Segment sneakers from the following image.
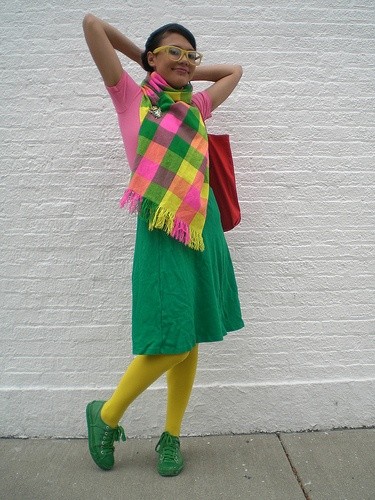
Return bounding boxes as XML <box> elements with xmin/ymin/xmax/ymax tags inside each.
<box><xmin>157</xmin><ymin>431</ymin><xmax>185</xmax><ymax>476</ymax></box>
<box><xmin>85</xmin><ymin>400</ymin><xmax>126</xmax><ymax>470</ymax></box>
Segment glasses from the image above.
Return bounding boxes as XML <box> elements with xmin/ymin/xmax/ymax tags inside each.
<box><xmin>153</xmin><ymin>45</ymin><xmax>203</xmax><ymax>66</ymax></box>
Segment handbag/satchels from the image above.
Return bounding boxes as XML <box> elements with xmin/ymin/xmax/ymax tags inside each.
<box><xmin>192</xmin><ymin>99</ymin><xmax>242</xmax><ymax>233</ymax></box>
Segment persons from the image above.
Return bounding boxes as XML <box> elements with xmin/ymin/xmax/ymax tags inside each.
<box><xmin>80</xmin><ymin>12</ymin><xmax>247</xmax><ymax>476</ymax></box>
